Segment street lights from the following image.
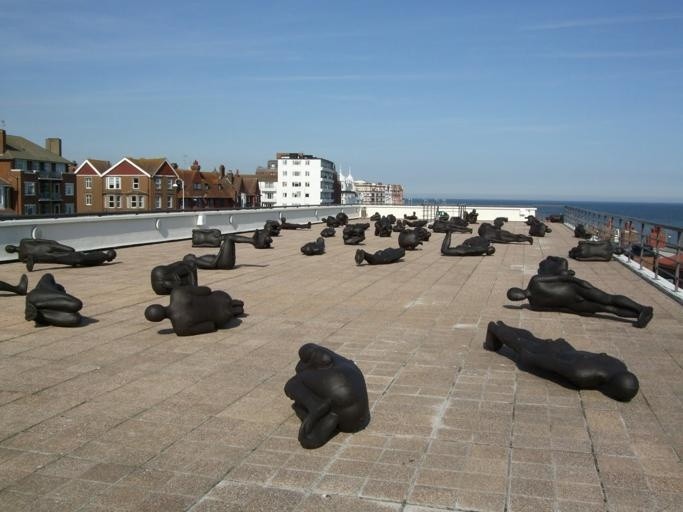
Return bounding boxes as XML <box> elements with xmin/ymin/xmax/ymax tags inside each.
<box><xmin>172</xmin><ymin>178</ymin><xmax>185</xmax><ymax>211</ymax></box>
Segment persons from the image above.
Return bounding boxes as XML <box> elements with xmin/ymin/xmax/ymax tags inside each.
<box><xmin>484</xmin><ymin>321</ymin><xmax>639</xmax><ymax>404</ymax></box>
<box><xmin>23</xmin><ymin>273</ymin><xmax>85</xmax><ymax>328</ymax></box>
<box><xmin>145</xmin><ymin>285</ymin><xmax>244</xmax><ymax>338</ymax></box>
<box><xmin>0</xmin><ymin>209</ymin><xmax>624</xmax><ymax>297</ymax></box>
<box><xmin>283</xmin><ymin>342</ymin><xmax>371</xmax><ymax>449</ymax></box>
<box><xmin>508</xmin><ymin>274</ymin><xmax>651</xmax><ymax>327</ymax></box>
